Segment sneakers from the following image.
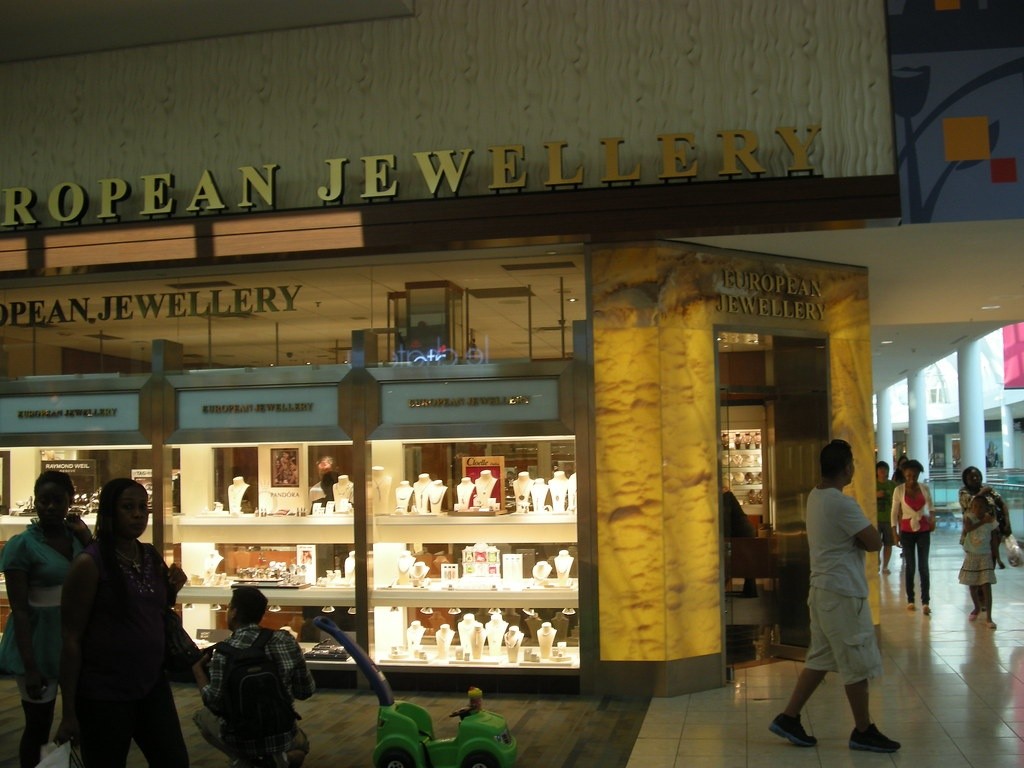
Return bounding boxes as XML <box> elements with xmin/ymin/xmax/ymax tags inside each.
<box><xmin>768</xmin><ymin>713</ymin><xmax>817</xmax><ymax>747</ymax></box>
<box><xmin>849</xmin><ymin>723</ymin><xmax>901</xmax><ymax>753</ymax></box>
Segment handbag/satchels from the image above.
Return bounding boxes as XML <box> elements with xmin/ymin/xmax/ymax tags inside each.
<box><xmin>34</xmin><ymin>736</ymin><xmax>85</xmax><ymax>768</ymax></box>
<box><xmin>990</xmin><ymin>490</ymin><xmax>1012</xmax><ymax>537</ymax></box>
<box><xmin>149</xmin><ymin>543</ymin><xmax>204</xmax><ymax>672</ymax></box>
<box><xmin>1005</xmin><ymin>534</ymin><xmax>1024</xmax><ymax>567</ymax></box>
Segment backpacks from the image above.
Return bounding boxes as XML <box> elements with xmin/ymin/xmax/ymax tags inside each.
<box><xmin>202</xmin><ymin>629</ymin><xmax>303</xmax><ymax>740</ymax></box>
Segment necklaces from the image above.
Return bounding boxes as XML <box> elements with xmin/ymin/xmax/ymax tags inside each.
<box><xmin>721</xmin><ymin>433</ymin><xmax>762</xmax><ymax>464</ymax></box>
<box><xmin>114</xmin><ymin>544</ymin><xmax>141</xmax><ymax>573</ymax></box>
<box><xmin>373</xmin><ymin>471</ymin><xmax>577</xmax><ymax>511</ymax></box>
<box><xmin>397</xmin><ymin>552</ymin><xmax>571</xmax><ymax>649</ymax></box>
<box><xmin>231</xmin><ymin>485</ymin><xmax>246</xmax><ymax>499</ymax></box>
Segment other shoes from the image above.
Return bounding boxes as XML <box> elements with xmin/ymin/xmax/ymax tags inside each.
<box><xmin>264</xmin><ymin>751</ymin><xmax>290</xmax><ymax>768</ymax></box>
<box><xmin>985</xmin><ymin>620</ymin><xmax>996</xmax><ymax>628</ymax></box>
<box><xmin>969</xmin><ymin>608</ymin><xmax>981</xmax><ymax>621</ymax></box>
<box><xmin>229</xmin><ymin>758</ymin><xmax>258</xmax><ymax>768</ymax></box>
<box><xmin>900</xmin><ymin>553</ymin><xmax>905</xmax><ymax>558</ymax></box>
<box><xmin>882</xmin><ymin>568</ymin><xmax>892</xmax><ymax>575</ymax></box>
<box><xmin>908</xmin><ymin>603</ymin><xmax>915</xmax><ymax>611</ymax></box>
<box><xmin>922</xmin><ymin>605</ymin><xmax>931</xmax><ymax>614</ymax></box>
<box><xmin>878</xmin><ymin>561</ymin><xmax>881</xmax><ymax>573</ymax></box>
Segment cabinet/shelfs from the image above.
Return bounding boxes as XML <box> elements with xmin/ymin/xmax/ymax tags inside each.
<box><xmin>0</xmin><ymin>435</ymin><xmax>580</xmax><ymax>676</ymax></box>
<box><xmin>720</xmin><ymin>427</ymin><xmax>765</xmax><ymax>513</ymax></box>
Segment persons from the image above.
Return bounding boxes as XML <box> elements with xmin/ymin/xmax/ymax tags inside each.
<box><xmin>958</xmin><ymin>495</ymin><xmax>1005</xmax><ymax>629</ymax></box>
<box><xmin>958</xmin><ymin>466</ymin><xmax>1012</xmax><ymax>611</ymax></box>
<box><xmin>767</xmin><ymin>439</ymin><xmax>902</xmax><ymax>753</ymax></box>
<box><xmin>0</xmin><ymin>469</ymin><xmax>93</xmax><ymax>768</ymax></box>
<box><xmin>191</xmin><ymin>586</ymin><xmax>315</xmax><ymax>768</ymax></box>
<box><xmin>986</xmin><ymin>441</ymin><xmax>998</xmax><ymax>468</ymax></box>
<box><xmin>876</xmin><ymin>460</ymin><xmax>899</xmax><ymax>575</ymax></box>
<box><xmin>309</xmin><ymin>473</ymin><xmax>351</xmax><ymax>578</ymax></box>
<box><xmin>892</xmin><ymin>455</ymin><xmax>909</xmax><ymax>483</ymax></box>
<box><xmin>54</xmin><ymin>477</ymin><xmax>190</xmax><ymax>768</ymax></box>
<box><xmin>722</xmin><ymin>490</ymin><xmax>754</xmax><ymax>536</ymax></box>
<box><xmin>891</xmin><ymin>459</ymin><xmax>936</xmax><ymax>614</ymax></box>
